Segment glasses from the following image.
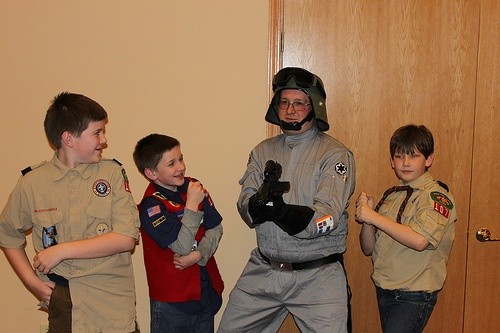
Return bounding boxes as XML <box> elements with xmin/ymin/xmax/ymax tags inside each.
<box><xmin>276</xmin><ymin>97</ymin><xmax>312</xmax><ymax>111</ymax></box>
<box><xmin>276</xmin><ymin>67</ymin><xmax>314</xmax><ymax>88</ymax></box>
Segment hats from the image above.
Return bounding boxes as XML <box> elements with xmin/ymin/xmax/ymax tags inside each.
<box><xmin>265</xmin><ymin>67</ymin><xmax>330</xmax><ymax>132</ymax></box>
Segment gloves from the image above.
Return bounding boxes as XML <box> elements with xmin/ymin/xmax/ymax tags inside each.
<box><xmin>249</xmin><ymin>160</ymin><xmax>290</xmax><ymax>222</ymax></box>
<box><xmin>253</xmin><ymin>191</ymin><xmax>315</xmax><ymax>236</ymax></box>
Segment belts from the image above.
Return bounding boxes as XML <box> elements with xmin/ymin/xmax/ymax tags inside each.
<box><xmin>258</xmin><ymin>248</ymin><xmax>342</xmax><ymax>271</ymax></box>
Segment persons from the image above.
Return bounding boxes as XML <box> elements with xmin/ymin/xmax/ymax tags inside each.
<box><xmin>355</xmin><ymin>125</ymin><xmax>456</xmax><ymax>333</ymax></box>
<box><xmin>212</xmin><ymin>68</ymin><xmax>356</xmax><ymax>333</ymax></box>
<box><xmin>0</xmin><ymin>91</ymin><xmax>140</xmax><ymax>333</ymax></box>
<box><xmin>133</xmin><ymin>134</ymin><xmax>226</xmax><ymax>333</ymax></box>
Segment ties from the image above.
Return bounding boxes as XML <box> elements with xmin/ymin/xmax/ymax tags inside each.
<box><xmin>374</xmin><ymin>185</ymin><xmax>413</xmax><ymax>226</ymax></box>
